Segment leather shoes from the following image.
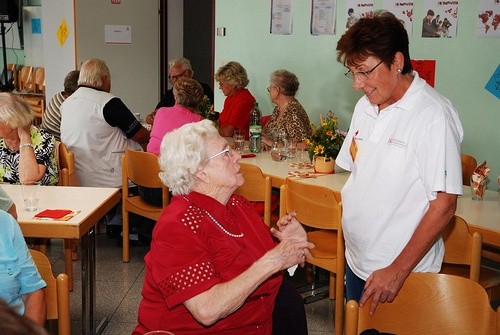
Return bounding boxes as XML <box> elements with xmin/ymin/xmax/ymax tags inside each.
<box><xmin>117</xmin><ymin>234</ymin><xmax>152</xmax><ymax>248</ymax></box>
<box><xmin>105</xmin><ymin>224</ymin><xmax>139</xmax><ymax>238</ymax></box>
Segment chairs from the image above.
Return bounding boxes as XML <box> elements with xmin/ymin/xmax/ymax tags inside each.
<box><xmin>22</xmin><ymin>249</ymin><xmax>70</xmax><ymax>335</ymax></box>
<box><xmin>121</xmin><ymin>148</ymin><xmax>169</xmax><ymax>263</ymax></box>
<box><xmin>7</xmin><ymin>64</ymin><xmax>45</xmax><ymax>127</ymax></box>
<box><xmin>56</xmin><ymin>142</ymin><xmax>78</xmax><ymax>186</ymax></box>
<box><xmin>238</xmin><ymin>163</ymin><xmax>500</xmax><ymax>335</ymax></box>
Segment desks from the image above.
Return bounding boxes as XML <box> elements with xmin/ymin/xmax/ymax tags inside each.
<box><xmin>0</xmin><ymin>184</ymin><xmax>122</xmax><ymax>335</ymax></box>
<box><xmin>225</xmin><ymin>137</ymin><xmax>500</xmax><ymax>260</ymax></box>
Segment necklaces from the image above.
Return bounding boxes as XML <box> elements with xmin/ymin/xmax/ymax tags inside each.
<box><xmin>184</xmin><ymin>196</ymin><xmax>244</xmax><ymax>237</ymax></box>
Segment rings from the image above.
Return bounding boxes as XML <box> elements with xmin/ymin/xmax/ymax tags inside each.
<box><xmin>303</xmin><ymin>249</ymin><xmax>305</xmax><ymax>256</ymax></box>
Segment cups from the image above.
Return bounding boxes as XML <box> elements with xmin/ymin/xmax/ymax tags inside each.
<box><xmin>232</xmin><ymin>129</ymin><xmax>245</xmax><ymax>154</ymax></box>
<box><xmin>21</xmin><ymin>180</ymin><xmax>40</xmax><ymax>212</ymax></box>
<box><xmin>0</xmin><ymin>182</ymin><xmax>10</xmax><ymax>197</ymax></box>
<box><xmin>296</xmin><ymin>151</ymin><xmax>308</xmax><ymax>163</ymax></box>
<box><xmin>470</xmin><ymin>176</ymin><xmax>488</xmax><ymax>201</ymax></box>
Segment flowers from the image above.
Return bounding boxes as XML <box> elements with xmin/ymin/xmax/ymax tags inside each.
<box><xmin>305</xmin><ymin>110</ymin><xmax>345</xmax><ymax>162</ymax></box>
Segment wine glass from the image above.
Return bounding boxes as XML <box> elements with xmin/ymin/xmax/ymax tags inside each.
<box><xmin>273</xmin><ymin>131</ymin><xmax>286</xmax><ymax>162</ymax></box>
<box><xmin>286</xmin><ymin>140</ymin><xmax>297</xmax><ymax>165</ymax></box>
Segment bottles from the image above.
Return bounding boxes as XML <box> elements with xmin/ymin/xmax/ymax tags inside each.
<box><xmin>249</xmin><ymin>103</ymin><xmax>262</xmax><ymax>153</ymax></box>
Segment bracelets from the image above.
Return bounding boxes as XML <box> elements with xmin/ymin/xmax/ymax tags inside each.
<box><xmin>19</xmin><ymin>144</ymin><xmax>34</xmax><ymax>149</ymax></box>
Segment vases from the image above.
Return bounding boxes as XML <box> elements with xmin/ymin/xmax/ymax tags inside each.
<box><xmin>315</xmin><ymin>157</ymin><xmax>335</xmax><ymax>173</ymax></box>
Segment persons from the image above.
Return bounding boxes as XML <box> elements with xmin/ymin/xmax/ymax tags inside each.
<box><xmin>346</xmin><ymin>8</ymin><xmax>359</xmax><ymax>28</ymax></box>
<box><xmin>131</xmin><ymin>119</ymin><xmax>315</xmax><ymax>335</ymax></box>
<box><xmin>60</xmin><ymin>58</ymin><xmax>150</xmax><ymax>247</ymax></box>
<box><xmin>336</xmin><ymin>9</ymin><xmax>463</xmax><ymax>335</ymax></box>
<box><xmin>145</xmin><ymin>57</ymin><xmax>214</xmax><ymax>125</ymax></box>
<box><xmin>261</xmin><ymin>69</ymin><xmax>313</xmax><ymax>150</ymax></box>
<box><xmin>0</xmin><ymin>184</ymin><xmax>17</xmax><ymax>220</ymax></box>
<box><xmin>215</xmin><ymin>61</ymin><xmax>256</xmax><ymax>141</ymax></box>
<box><xmin>0</xmin><ymin>209</ymin><xmax>48</xmax><ymax>326</ymax></box>
<box><xmin>442</xmin><ymin>18</ymin><xmax>453</xmax><ymax>29</ymax></box>
<box><xmin>41</xmin><ymin>70</ymin><xmax>80</xmax><ymax>141</ymax></box>
<box><xmin>0</xmin><ymin>92</ymin><xmax>59</xmax><ymax>248</ymax></box>
<box><xmin>0</xmin><ymin>296</ymin><xmax>48</xmax><ymax>335</ymax></box>
<box><xmin>422</xmin><ymin>9</ymin><xmax>441</xmax><ymax>38</ymax></box>
<box><xmin>138</xmin><ymin>78</ymin><xmax>206</xmax><ymax>205</ymax></box>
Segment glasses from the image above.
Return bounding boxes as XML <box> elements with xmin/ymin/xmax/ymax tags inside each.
<box><xmin>219</xmin><ymin>81</ymin><xmax>227</xmax><ymax>86</ymax></box>
<box><xmin>267</xmin><ymin>86</ymin><xmax>279</xmax><ymax>92</ymax></box>
<box><xmin>344</xmin><ymin>53</ymin><xmax>388</xmax><ymax>80</ymax></box>
<box><xmin>202</xmin><ymin>146</ymin><xmax>233</xmax><ymax>162</ymax></box>
<box><xmin>169</xmin><ymin>69</ymin><xmax>189</xmax><ymax>81</ymax></box>
